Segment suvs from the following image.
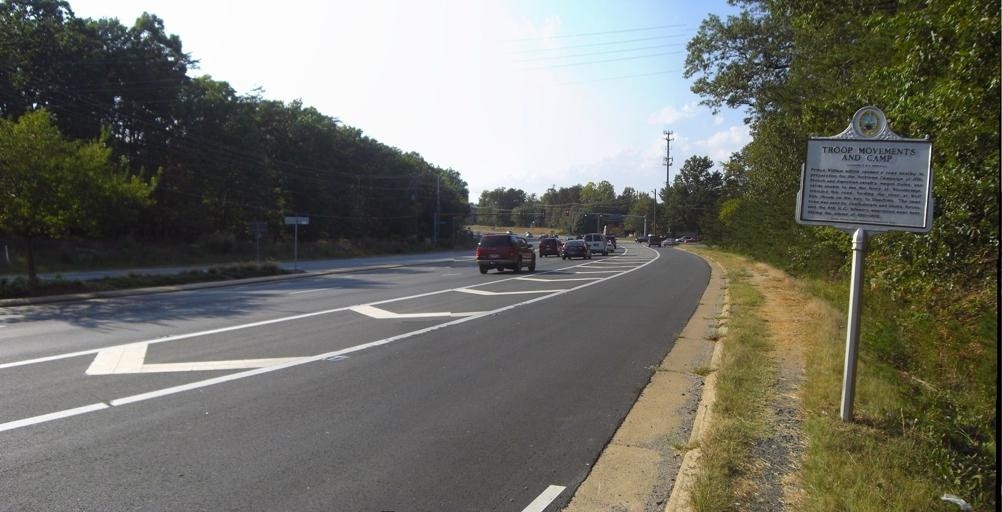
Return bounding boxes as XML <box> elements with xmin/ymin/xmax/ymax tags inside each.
<box><xmin>476</xmin><ymin>232</ymin><xmax>537</xmax><ymax>275</ymax></box>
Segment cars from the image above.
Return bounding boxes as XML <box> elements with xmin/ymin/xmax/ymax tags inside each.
<box><xmin>633</xmin><ymin>232</ymin><xmax>698</xmax><ymax>249</ymax></box>
<box><xmin>504</xmin><ymin>229</ymin><xmax>617</xmax><ymax>261</ymax></box>
